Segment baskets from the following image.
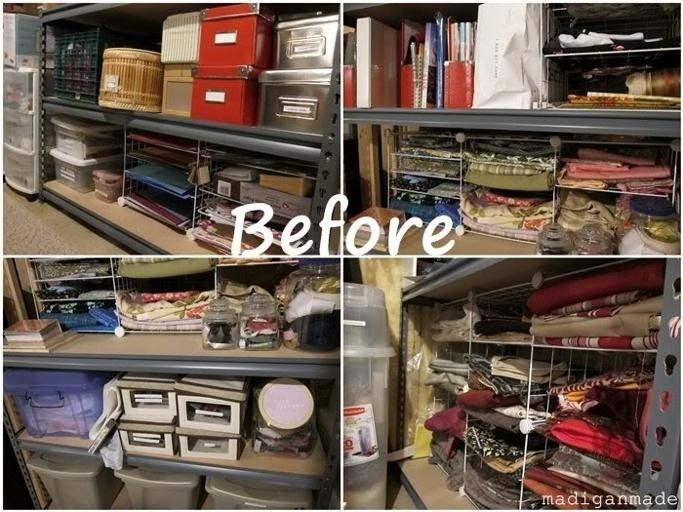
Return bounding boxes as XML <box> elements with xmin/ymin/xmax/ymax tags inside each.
<box><xmin>55</xmin><ymin>28</ymin><xmax>110</xmax><ymax>105</ymax></box>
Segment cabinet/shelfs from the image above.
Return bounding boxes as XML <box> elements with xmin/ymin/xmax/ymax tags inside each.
<box><xmin>3</xmin><ymin>3</ymin><xmax>681</xmax><ymax>510</ymax></box>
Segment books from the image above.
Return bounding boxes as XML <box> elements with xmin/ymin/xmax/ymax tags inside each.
<box><xmin>408</xmin><ymin>17</ymin><xmax>475</xmax><ymax>111</ymax></box>
<box><xmin>3</xmin><ymin>317</ymin><xmax>78</xmax><ymax>352</ymax></box>
